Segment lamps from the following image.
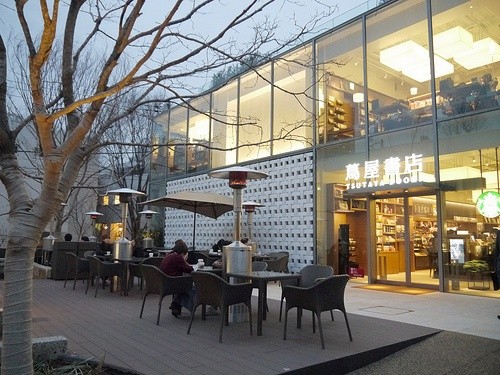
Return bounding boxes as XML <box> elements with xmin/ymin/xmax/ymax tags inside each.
<box><xmin>353</xmin><ymin>93</ymin><xmax>364</xmax><ymax>103</ymax></box>
<box><xmin>379</xmin><ymin>25</ymin><xmax>500</xmax><ymax>83</ymax></box>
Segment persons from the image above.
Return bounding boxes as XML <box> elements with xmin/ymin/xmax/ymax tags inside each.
<box><xmin>422</xmin><ymin>231</ymin><xmax>437</xmax><ymax>269</ymax></box>
<box><xmin>241</xmin><ymin>238</ymin><xmax>249</xmax><ymax>244</ymax></box>
<box><xmin>209</xmin><ymin>238</ymin><xmax>226</xmax><ymax>256</ymax></box>
<box><xmin>160</xmin><ymin>242</ymin><xmax>204</xmax><ymax>319</ymax></box>
<box><xmin>65</xmin><ymin>233</ymin><xmax>90</xmax><ymax>242</ymax></box>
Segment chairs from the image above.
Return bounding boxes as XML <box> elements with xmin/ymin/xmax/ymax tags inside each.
<box><xmin>34</xmin><ymin>241</ymin><xmax>353</xmax><ymax>350</ymax></box>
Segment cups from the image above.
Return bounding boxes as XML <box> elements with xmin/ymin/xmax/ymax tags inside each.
<box><xmin>198</xmin><ymin>259</ymin><xmax>203</xmax><ymax>268</ymax></box>
<box><xmin>218</xmin><ymin>250</ymin><xmax>221</xmax><ymax>255</ymax></box>
<box><xmin>210</xmin><ymin>249</ymin><xmax>213</xmax><ymax>254</ymax></box>
<box><xmin>149</xmin><ymin>253</ymin><xmax>153</xmax><ymax>257</ymax></box>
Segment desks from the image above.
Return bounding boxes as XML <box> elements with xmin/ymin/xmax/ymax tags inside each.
<box><xmin>370</xmin><ymin>103</ymin><xmax>411</xmax><ymax>131</ymax></box>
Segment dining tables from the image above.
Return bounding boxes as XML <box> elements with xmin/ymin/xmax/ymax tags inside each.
<box><xmin>190</xmin><ymin>265</ymin><xmax>222</xmax><ymax>321</ymax></box>
<box><xmin>228</xmin><ymin>273</ymin><xmax>302</xmax><ymax>337</ymax></box>
<box><xmin>116</xmin><ymin>258</ymin><xmax>143</xmax><ymax>296</ymax></box>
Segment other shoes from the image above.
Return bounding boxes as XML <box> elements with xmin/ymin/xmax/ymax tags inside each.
<box><xmin>168</xmin><ymin>302</ymin><xmax>181</xmax><ymax>318</ymax></box>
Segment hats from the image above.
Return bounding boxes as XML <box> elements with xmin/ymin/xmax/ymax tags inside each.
<box><xmin>171</xmin><ymin>239</ymin><xmax>189</xmax><ymax>253</ymax></box>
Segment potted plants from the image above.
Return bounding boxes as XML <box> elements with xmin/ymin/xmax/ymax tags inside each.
<box><xmin>463</xmin><ymin>259</ymin><xmax>494</xmax><ymax>290</ymax></box>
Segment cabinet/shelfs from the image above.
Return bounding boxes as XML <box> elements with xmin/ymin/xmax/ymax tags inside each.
<box><xmin>408</xmin><ymin>91</ymin><xmax>452</xmax><ymax>124</ymax></box>
<box><xmin>375</xmin><ymin>198</ymin><xmax>499</xmax><ymax>280</ymax></box>
<box><xmin>329</xmin><ymin>99</ymin><xmax>378</xmax><ymax>141</ymax></box>
